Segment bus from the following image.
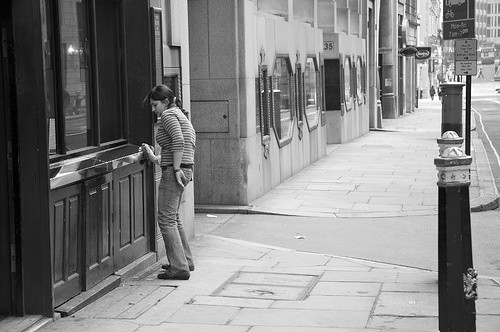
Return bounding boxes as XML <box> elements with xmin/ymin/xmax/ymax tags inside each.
<box><xmin>481</xmin><ymin>42</ymin><xmax>496</xmax><ymax>64</ymax></box>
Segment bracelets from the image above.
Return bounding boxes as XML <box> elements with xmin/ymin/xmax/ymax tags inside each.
<box><xmin>174</xmin><ymin>169</ymin><xmax>181</xmax><ymax>173</ymax></box>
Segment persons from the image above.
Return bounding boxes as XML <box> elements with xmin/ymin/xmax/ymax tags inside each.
<box><xmin>141</xmin><ymin>83</ymin><xmax>196</xmax><ymax>280</ymax></box>
<box><xmin>429</xmin><ymin>64</ymin><xmax>485</xmax><ymax>100</ymax></box>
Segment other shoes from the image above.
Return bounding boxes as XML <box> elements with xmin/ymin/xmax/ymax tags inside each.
<box><xmin>157</xmin><ymin>271</ymin><xmax>190</xmax><ymax>280</ymax></box>
<box><xmin>161</xmin><ymin>264</ymin><xmax>194</xmax><ymax>271</ymax></box>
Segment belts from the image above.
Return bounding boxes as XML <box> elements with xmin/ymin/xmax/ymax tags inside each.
<box><xmin>168</xmin><ymin>165</ymin><xmax>192</xmax><ymax>168</ymax></box>
